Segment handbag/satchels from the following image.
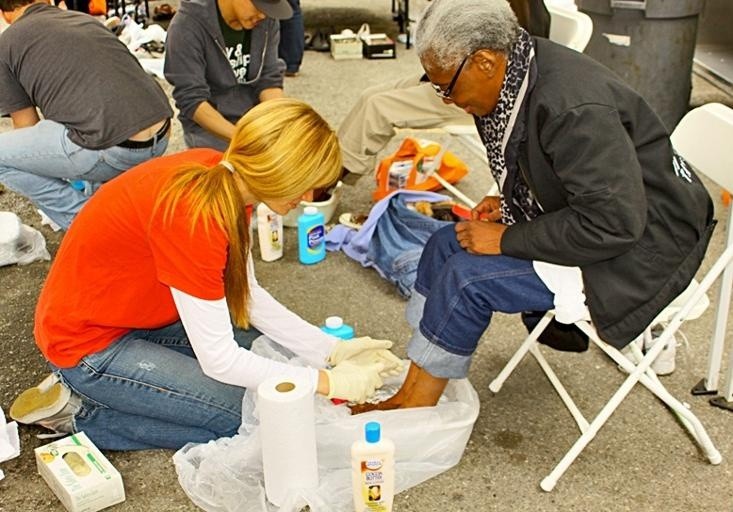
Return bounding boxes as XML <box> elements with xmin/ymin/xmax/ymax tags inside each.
<box><xmin>371</xmin><ymin>137</ymin><xmax>475</xmax><ymax>299</ymax></box>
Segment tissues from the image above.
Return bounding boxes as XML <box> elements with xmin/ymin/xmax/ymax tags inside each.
<box><xmin>33</xmin><ymin>430</ymin><xmax>125</xmax><ymax>512</ymax></box>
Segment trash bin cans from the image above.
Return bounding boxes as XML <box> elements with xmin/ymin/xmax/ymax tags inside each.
<box><xmin>567</xmin><ymin>0</ymin><xmax>706</xmax><ymax>137</ymax></box>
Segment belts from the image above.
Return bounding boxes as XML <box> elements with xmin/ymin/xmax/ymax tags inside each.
<box><xmin>118</xmin><ymin>118</ymin><xmax>171</xmax><ymax>150</ymax></box>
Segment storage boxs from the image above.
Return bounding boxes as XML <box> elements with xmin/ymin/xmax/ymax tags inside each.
<box><xmin>359</xmin><ymin>33</ymin><xmax>396</xmax><ymax>59</ymax></box>
<box><xmin>329</xmin><ymin>34</ymin><xmax>363</xmax><ymax>60</ymax></box>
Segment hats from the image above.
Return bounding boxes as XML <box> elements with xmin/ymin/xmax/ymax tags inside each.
<box><xmin>251</xmin><ymin>1</ymin><xmax>296</xmax><ymax>19</ymax></box>
<box><xmin>0</xmin><ymin>212</ymin><xmax>46</xmax><ymax>267</ymax></box>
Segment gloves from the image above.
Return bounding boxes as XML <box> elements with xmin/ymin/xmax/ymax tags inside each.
<box><xmin>315</xmin><ymin>335</ymin><xmax>402</xmax><ymax>404</ymax></box>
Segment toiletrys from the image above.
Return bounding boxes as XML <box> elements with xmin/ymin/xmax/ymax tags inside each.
<box><xmin>257</xmin><ymin>202</ymin><xmax>283</xmax><ymax>262</ymax></box>
<box><xmin>349</xmin><ymin>422</ymin><xmax>394</xmax><ymax>512</ymax></box>
<box><xmin>0</xmin><ymin>211</ymin><xmax>20</xmax><ymax>243</ymax></box>
<box><xmin>297</xmin><ymin>207</ymin><xmax>326</xmax><ymax>265</ymax></box>
<box><xmin>320</xmin><ymin>316</ymin><xmax>354</xmax><ymax>340</ymax></box>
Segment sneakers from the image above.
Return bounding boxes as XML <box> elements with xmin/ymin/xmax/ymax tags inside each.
<box><xmin>617</xmin><ymin>323</ymin><xmax>677</xmax><ymax>378</ymax></box>
<box><xmin>10</xmin><ymin>380</ymin><xmax>80</xmax><ymax>428</ymax></box>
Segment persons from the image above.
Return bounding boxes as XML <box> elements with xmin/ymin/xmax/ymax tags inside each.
<box><xmin>8</xmin><ymin>98</ymin><xmax>408</xmax><ymax>452</ymax></box>
<box><xmin>350</xmin><ymin>1</ymin><xmax>720</xmax><ymax>419</ymax></box>
<box><xmin>163</xmin><ymin>1</ymin><xmax>293</xmax><ymax>154</ymax></box>
<box><xmin>279</xmin><ymin>0</ymin><xmax>305</xmax><ymax>76</ymax></box>
<box><xmin>310</xmin><ymin>1</ymin><xmax>550</xmax><ymax>200</ymax></box>
<box><xmin>0</xmin><ymin>0</ymin><xmax>176</xmax><ymax>232</ymax></box>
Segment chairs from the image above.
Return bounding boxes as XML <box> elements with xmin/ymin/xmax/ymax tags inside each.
<box><xmin>420</xmin><ymin>3</ymin><xmax>593</xmax><ymax>208</ymax></box>
<box><xmin>488</xmin><ymin>101</ymin><xmax>733</xmax><ymax>493</ymax></box>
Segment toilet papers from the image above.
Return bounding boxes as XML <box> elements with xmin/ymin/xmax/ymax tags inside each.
<box><xmin>257</xmin><ymin>375</ymin><xmax>319</xmax><ymax>507</ymax></box>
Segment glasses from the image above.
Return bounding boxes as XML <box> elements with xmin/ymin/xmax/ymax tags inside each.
<box><xmin>431</xmin><ymin>55</ymin><xmax>470</xmax><ymax>99</ymax></box>
